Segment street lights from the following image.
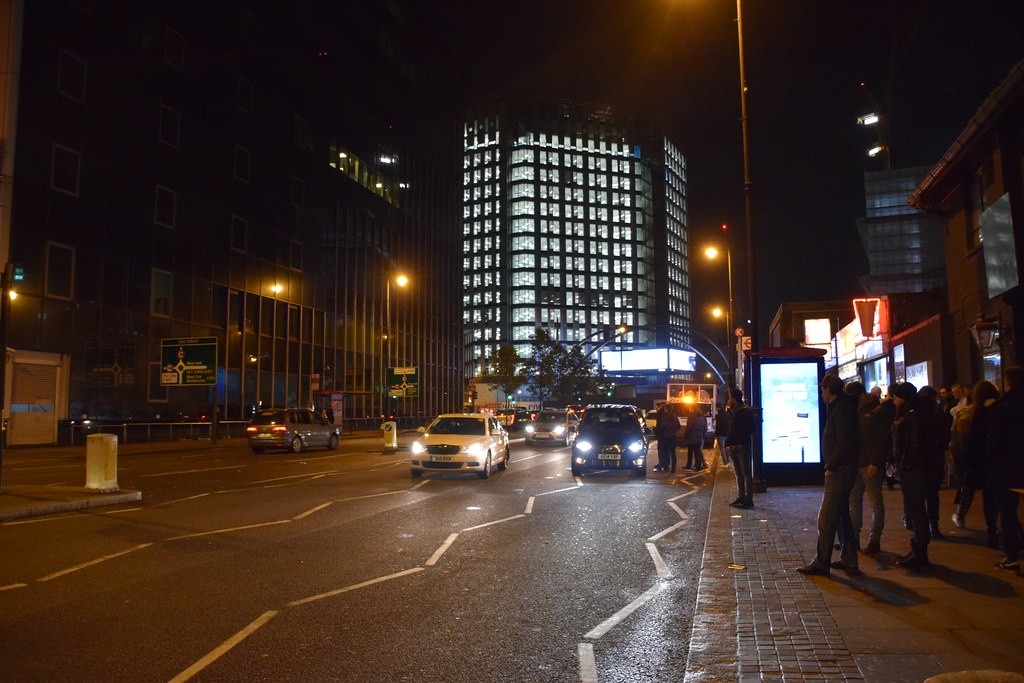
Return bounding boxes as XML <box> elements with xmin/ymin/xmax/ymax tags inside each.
<box><xmin>703</xmin><ymin>239</ymin><xmax>738</xmax><ymax>400</ymax></box>
<box><xmin>507</xmin><ymin>395</ymin><xmax>515</xmax><ymax>409</ymax></box>
<box><xmin>385</xmin><ymin>272</ymin><xmax>410</xmax><ymax>411</ymax></box>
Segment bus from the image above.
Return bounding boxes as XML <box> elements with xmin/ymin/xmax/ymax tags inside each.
<box><xmin>666</xmin><ymin>383</ymin><xmax>718</xmax><ymax>434</ymax></box>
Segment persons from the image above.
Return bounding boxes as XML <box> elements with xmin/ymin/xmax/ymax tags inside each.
<box><xmin>682</xmin><ymin>403</ymin><xmax>707</xmax><ymax>471</ymax></box>
<box><xmin>798</xmin><ymin>366</ymin><xmax>1024</xmax><ymax>575</ymax></box>
<box><xmin>715</xmin><ymin>389</ymin><xmax>754</xmax><ymax>507</ymax></box>
<box><xmin>654</xmin><ymin>402</ymin><xmax>681</xmax><ymax>473</ymax></box>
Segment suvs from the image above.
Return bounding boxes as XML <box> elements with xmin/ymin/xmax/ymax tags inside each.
<box><xmin>664</xmin><ymin>402</ymin><xmax>715</xmax><ymax>446</ymax></box>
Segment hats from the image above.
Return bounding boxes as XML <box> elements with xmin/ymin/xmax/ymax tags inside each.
<box><xmin>893</xmin><ymin>382</ymin><xmax>917</xmax><ymax>401</ymax></box>
<box><xmin>819</xmin><ymin>374</ymin><xmax>844</xmax><ymax>389</ymax></box>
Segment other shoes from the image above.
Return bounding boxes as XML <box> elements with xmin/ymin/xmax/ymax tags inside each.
<box><xmin>729</xmin><ymin>498</ymin><xmax>753</xmax><ymax>509</ymax></box>
<box><xmin>930</xmin><ymin>529</ymin><xmax>944</xmax><ymax>540</ymax></box>
<box><xmin>834</xmin><ymin>543</ymin><xmax>841</xmax><ymax>548</ymax></box>
<box><xmin>899</xmin><ymin>516</ymin><xmax>912</xmax><ymax>528</ymax></box>
<box><xmin>951</xmin><ymin>512</ymin><xmax>965</xmax><ymax>527</ymax></box>
<box><xmin>993</xmin><ymin>560</ymin><xmax>1021</xmax><ymax>572</ymax></box>
<box><xmin>831</xmin><ymin>559</ymin><xmax>858</xmax><ymax>570</ymax></box>
<box><xmin>861</xmin><ymin>543</ymin><xmax>880</xmax><ymax>554</ymax></box>
<box><xmin>796</xmin><ymin>561</ymin><xmax>830</xmax><ymax>575</ymax></box>
<box><xmin>682</xmin><ymin>462</ymin><xmax>691</xmax><ymax>469</ymax></box>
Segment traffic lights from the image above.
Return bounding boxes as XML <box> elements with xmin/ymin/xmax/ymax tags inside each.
<box><xmin>474</xmin><ymin>391</ymin><xmax>477</xmax><ymax>399</ymax></box>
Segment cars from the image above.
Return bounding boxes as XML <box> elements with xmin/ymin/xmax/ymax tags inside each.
<box><xmin>178</xmin><ymin>404</ymin><xmax>220</xmax><ymax>430</ymax></box>
<box><xmin>572</xmin><ymin>404</ymin><xmax>652</xmax><ymax>482</ymax></box>
<box><xmin>646</xmin><ymin>409</ymin><xmax>657</xmax><ymax>429</ymax></box>
<box><xmin>245</xmin><ymin>407</ymin><xmax>342</xmax><ymax>454</ymax></box>
<box><xmin>409</xmin><ymin>412</ymin><xmax>511</xmax><ymax>481</ymax></box>
<box><xmin>525</xmin><ymin>410</ymin><xmax>580</xmax><ymax>447</ymax></box>
<box><xmin>480</xmin><ymin>407</ymin><xmax>527</xmax><ymax>426</ymax></box>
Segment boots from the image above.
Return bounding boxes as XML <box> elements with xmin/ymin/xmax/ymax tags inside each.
<box><xmin>896</xmin><ymin>539</ymin><xmax>929</xmax><ymax>568</ymax></box>
